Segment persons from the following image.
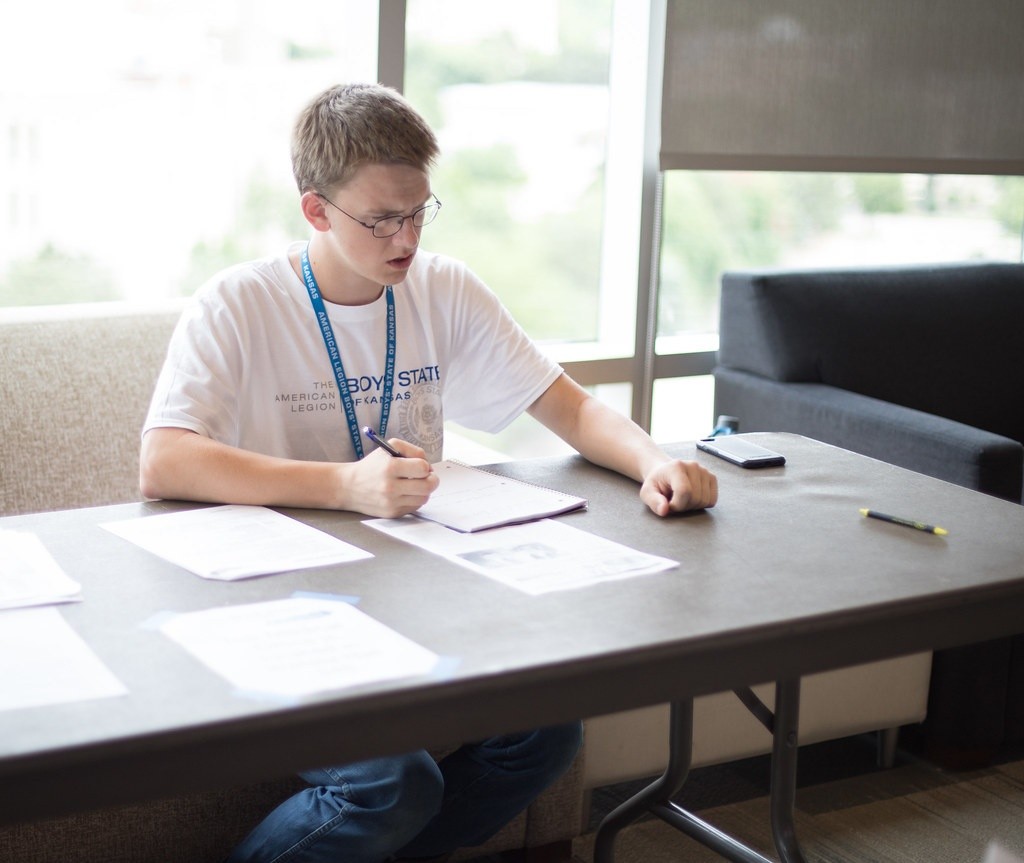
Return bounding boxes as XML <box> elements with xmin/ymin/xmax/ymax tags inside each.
<box><xmin>137</xmin><ymin>80</ymin><xmax>722</xmax><ymax>863</ymax></box>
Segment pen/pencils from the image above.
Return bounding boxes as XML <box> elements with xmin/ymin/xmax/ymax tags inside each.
<box><xmin>362</xmin><ymin>425</ymin><xmax>404</xmax><ymax>458</ymax></box>
<box><xmin>858</xmin><ymin>505</ymin><xmax>949</xmax><ymax>540</ymax></box>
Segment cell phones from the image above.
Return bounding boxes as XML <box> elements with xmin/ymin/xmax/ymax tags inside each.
<box><xmin>697</xmin><ymin>435</ymin><xmax>786</xmax><ymax>468</ymax></box>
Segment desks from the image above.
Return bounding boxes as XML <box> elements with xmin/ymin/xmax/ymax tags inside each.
<box><xmin>0</xmin><ymin>429</ymin><xmax>1024</xmax><ymax>863</ymax></box>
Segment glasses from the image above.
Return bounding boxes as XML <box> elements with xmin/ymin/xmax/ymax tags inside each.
<box><xmin>315</xmin><ymin>192</ymin><xmax>443</xmax><ymax>238</ymax></box>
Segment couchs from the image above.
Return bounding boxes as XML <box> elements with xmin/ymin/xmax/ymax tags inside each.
<box><xmin>712</xmin><ymin>261</ymin><xmax>1024</xmax><ymax>772</ymax></box>
<box><xmin>0</xmin><ymin>301</ymin><xmax>584</xmax><ymax>863</ymax></box>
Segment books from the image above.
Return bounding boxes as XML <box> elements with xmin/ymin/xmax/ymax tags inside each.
<box><xmin>408</xmin><ymin>456</ymin><xmax>591</xmax><ymax>536</ymax></box>
